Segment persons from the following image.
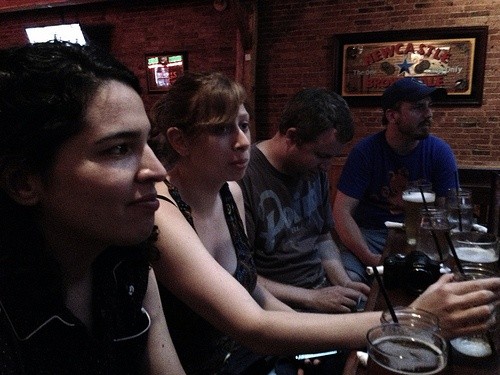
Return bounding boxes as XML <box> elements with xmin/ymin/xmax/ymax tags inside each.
<box><xmin>333</xmin><ymin>76</ymin><xmax>460</xmax><ymax>375</ymax></box>
<box><xmin>237</xmin><ymin>84</ymin><xmax>371</xmax><ymax>314</ymax></box>
<box><xmin>0</xmin><ymin>39</ymin><xmax>186</xmax><ymax>375</ymax></box>
<box><xmin>142</xmin><ymin>67</ymin><xmax>500</xmax><ymax>375</ymax></box>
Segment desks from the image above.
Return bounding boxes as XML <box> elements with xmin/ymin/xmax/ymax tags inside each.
<box><xmin>346</xmin><ymin>229</ymin><xmax>500</xmax><ymax>375</ymax></box>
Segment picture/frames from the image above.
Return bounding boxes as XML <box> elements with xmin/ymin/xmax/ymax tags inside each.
<box><xmin>332</xmin><ymin>26</ymin><xmax>488</xmax><ymax>108</ymax></box>
<box><xmin>145</xmin><ymin>51</ymin><xmax>187</xmax><ymax>93</ymax></box>
